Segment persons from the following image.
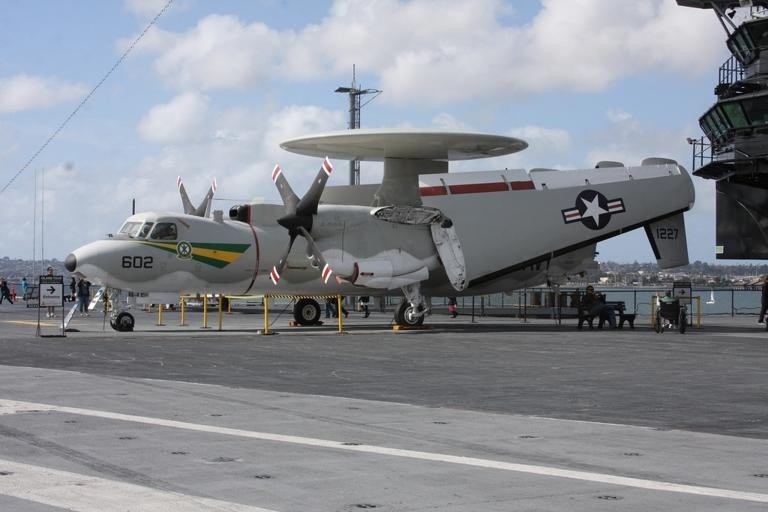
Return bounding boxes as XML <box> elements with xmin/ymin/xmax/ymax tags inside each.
<box><xmin>446</xmin><ymin>296</ymin><xmax>458</xmax><ymax>318</ymax></box>
<box><xmin>324</xmin><ymin>297</ymin><xmax>336</xmax><ymax>318</ymax></box>
<box><xmin>78</xmin><ymin>278</ymin><xmax>91</xmax><ymax>317</ymax></box>
<box><xmin>659</xmin><ymin>289</ymin><xmax>674</xmax><ymax>328</ymax></box>
<box><xmin>358</xmin><ymin>296</ymin><xmax>371</xmax><ymax>318</ymax></box>
<box><xmin>69</xmin><ymin>276</ymin><xmax>76</xmax><ymax>301</ymax></box>
<box><xmin>45</xmin><ymin>266</ymin><xmax>57</xmax><ymax>318</ymax></box>
<box><xmin>582</xmin><ymin>285</ymin><xmax>617</xmax><ymax>329</ymax></box>
<box><xmin>0</xmin><ymin>276</ymin><xmax>13</xmax><ymax>305</ymax></box>
<box><xmin>21</xmin><ymin>277</ymin><xmax>28</xmax><ymax>300</ymax></box>
<box><xmin>758</xmin><ymin>275</ymin><xmax>768</xmax><ymax>325</ymax></box>
<box><xmin>331</xmin><ymin>297</ymin><xmax>349</xmax><ymax>318</ymax></box>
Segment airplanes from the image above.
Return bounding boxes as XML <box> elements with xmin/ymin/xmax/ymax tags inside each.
<box><xmin>65</xmin><ymin>129</ymin><xmax>695</xmax><ymax>331</ymax></box>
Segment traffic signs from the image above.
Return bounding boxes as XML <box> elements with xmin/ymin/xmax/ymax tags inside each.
<box><xmin>39</xmin><ymin>275</ymin><xmax>64</xmax><ymax>308</ymax></box>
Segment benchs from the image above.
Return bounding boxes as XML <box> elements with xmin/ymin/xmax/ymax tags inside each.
<box><xmin>577</xmin><ymin>301</ymin><xmax>636</xmax><ymax>329</ymax></box>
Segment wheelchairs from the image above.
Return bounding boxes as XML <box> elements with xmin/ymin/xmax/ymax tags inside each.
<box><xmin>656</xmin><ymin>296</ymin><xmax>687</xmax><ymax>334</ymax></box>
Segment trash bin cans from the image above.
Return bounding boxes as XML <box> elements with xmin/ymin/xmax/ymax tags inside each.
<box><xmin>545</xmin><ymin>292</ymin><xmax>567</xmax><ymax>307</ymax></box>
<box><xmin>530</xmin><ymin>291</ymin><xmax>542</xmax><ymax>305</ymax></box>
<box><xmin>221</xmin><ymin>299</ymin><xmax>229</xmax><ymax>312</ymax></box>
